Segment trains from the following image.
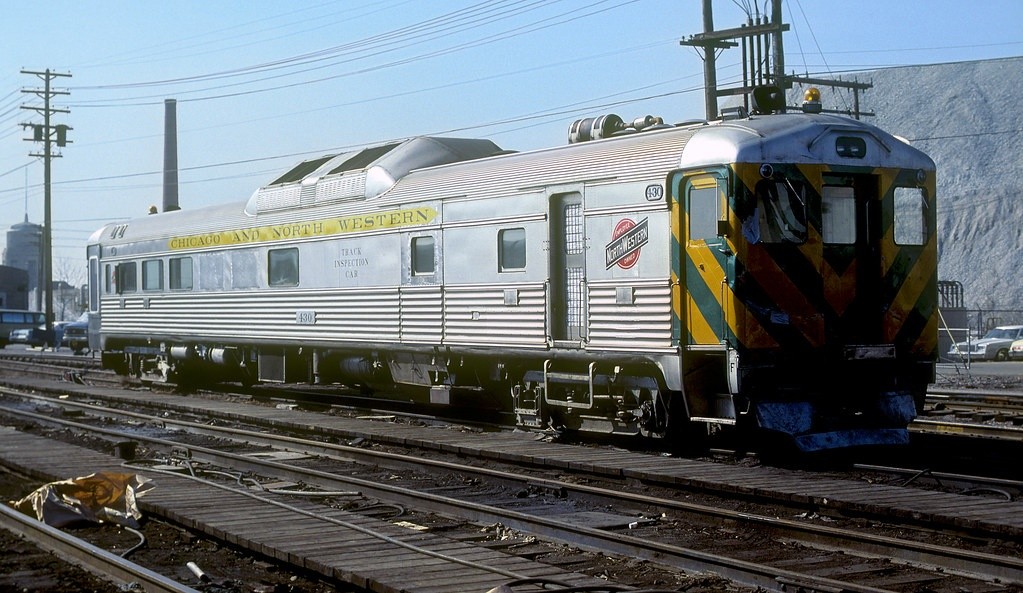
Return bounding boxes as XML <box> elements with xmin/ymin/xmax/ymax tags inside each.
<box><xmin>87</xmin><ymin>85</ymin><xmax>940</xmax><ymax>453</ymax></box>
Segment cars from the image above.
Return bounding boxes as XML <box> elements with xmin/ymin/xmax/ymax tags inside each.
<box><xmin>947</xmin><ymin>325</ymin><xmax>1023</xmax><ymax>362</ymax></box>
<box><xmin>9</xmin><ymin>321</ymin><xmax>75</xmax><ymax>348</ymax></box>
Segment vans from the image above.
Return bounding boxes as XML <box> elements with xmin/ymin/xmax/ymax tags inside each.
<box><xmin>0</xmin><ymin>309</ymin><xmax>54</xmax><ymax>349</ymax></box>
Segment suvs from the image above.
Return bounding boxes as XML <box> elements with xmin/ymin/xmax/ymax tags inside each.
<box><xmin>62</xmin><ymin>312</ymin><xmax>89</xmax><ymax>351</ymax></box>
